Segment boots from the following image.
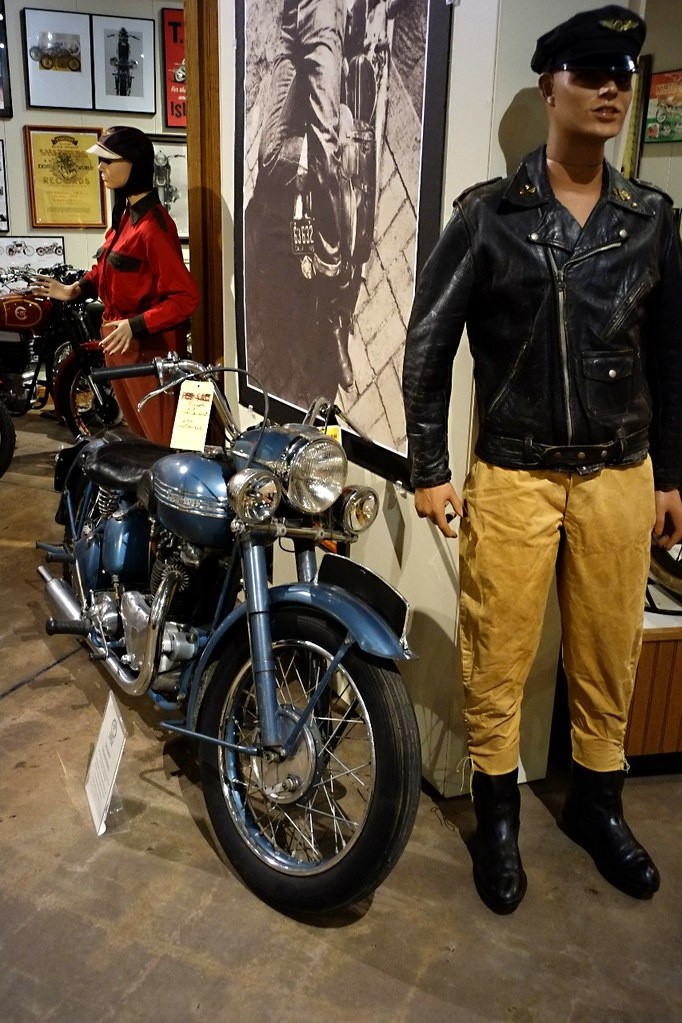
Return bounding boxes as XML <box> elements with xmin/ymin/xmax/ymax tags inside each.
<box><xmin>472</xmin><ymin>767</ymin><xmax>528</xmax><ymax>915</ymax></box>
<box><xmin>557</xmin><ymin>759</ymin><xmax>660</xmax><ymax>894</ymax></box>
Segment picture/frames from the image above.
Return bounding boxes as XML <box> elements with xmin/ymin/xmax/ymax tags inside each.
<box><xmin>22</xmin><ymin>125</ymin><xmax>108</xmax><ymax>229</ymax></box>
<box><xmin>20</xmin><ymin>6</ymin><xmax>156</xmax><ymax>114</ymax></box>
<box><xmin>145</xmin><ymin>133</ymin><xmax>190</xmax><ymax>245</ymax></box>
<box><xmin>161</xmin><ymin>7</ymin><xmax>186</xmax><ymax>128</ymax></box>
<box><xmin>0</xmin><ymin>0</ymin><xmax>14</xmax><ymax>119</ymax></box>
<box><xmin>0</xmin><ymin>139</ymin><xmax>9</xmax><ymax>233</ymax></box>
<box><xmin>0</xmin><ymin>235</ymin><xmax>65</xmax><ymax>294</ymax></box>
<box><xmin>644</xmin><ymin>68</ymin><xmax>682</xmax><ymax>143</ymax></box>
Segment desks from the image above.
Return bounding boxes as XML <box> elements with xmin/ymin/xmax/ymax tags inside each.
<box><xmin>623</xmin><ymin>545</ymin><xmax>682</xmax><ymax>780</ymax></box>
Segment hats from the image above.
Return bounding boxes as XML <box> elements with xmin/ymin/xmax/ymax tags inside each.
<box><xmin>86</xmin><ymin>126</ymin><xmax>155</xmax><ymax>160</ymax></box>
<box><xmin>531</xmin><ymin>4</ymin><xmax>647</xmax><ymax>77</ymax></box>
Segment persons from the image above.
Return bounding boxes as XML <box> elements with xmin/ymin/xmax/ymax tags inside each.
<box><xmin>404</xmin><ymin>7</ymin><xmax>682</xmax><ymax>910</ymax></box>
<box><xmin>29</xmin><ymin>126</ymin><xmax>203</xmax><ymax>447</ymax></box>
<box><xmin>257</xmin><ymin>0</ymin><xmax>346</xmax><ymax>287</ymax></box>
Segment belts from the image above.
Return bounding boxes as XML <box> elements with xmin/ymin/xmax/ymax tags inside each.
<box><xmin>539</xmin><ymin>449</ymin><xmax>648</xmax><ymax>475</ymax></box>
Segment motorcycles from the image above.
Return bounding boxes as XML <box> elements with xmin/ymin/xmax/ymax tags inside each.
<box><xmin>36</xmin><ymin>353</ymin><xmax>424</xmax><ymax>915</ymax></box>
<box><xmin>30</xmin><ymin>42</ymin><xmax>82</xmax><ymax>72</ymax></box>
<box><xmin>0</xmin><ymin>241</ymin><xmax>63</xmax><ymax>294</ymax></box>
<box><xmin>155</xmin><ymin>149</ymin><xmax>185</xmax><ymax>213</ymax></box>
<box><xmin>0</xmin><ymin>266</ymin><xmax>132</xmax><ymax>440</ymax></box>
<box><xmin>107</xmin><ymin>28</ymin><xmax>141</xmax><ymax>97</ymax></box>
<box><xmin>276</xmin><ymin>0</ymin><xmax>398</xmax><ymax>299</ymax></box>
